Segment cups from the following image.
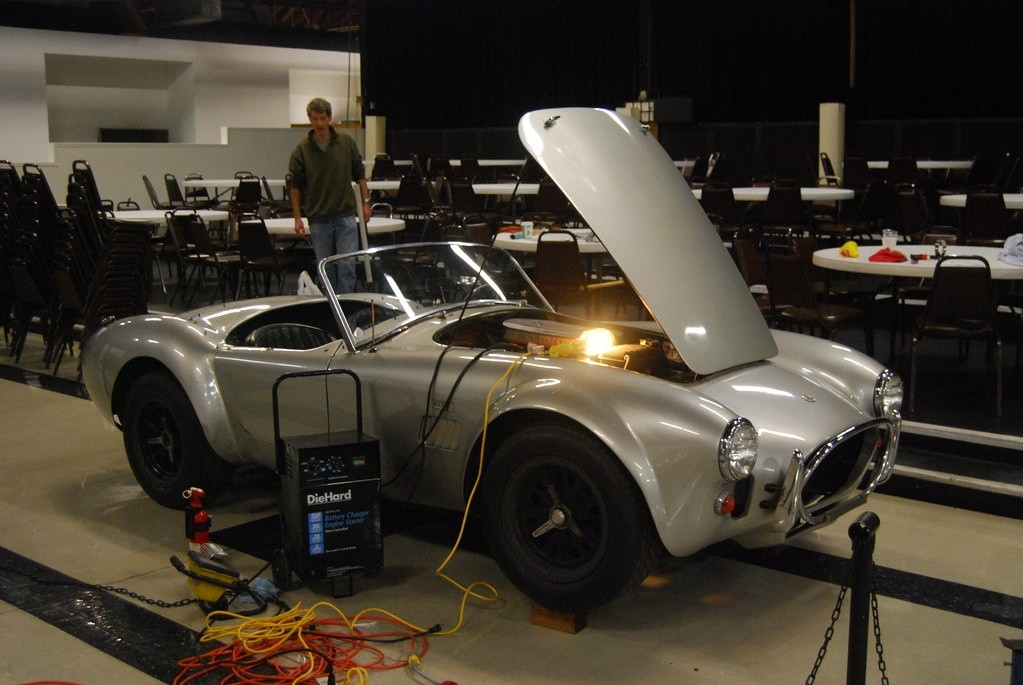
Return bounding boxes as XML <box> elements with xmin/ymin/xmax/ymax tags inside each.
<box><xmin>882</xmin><ymin>228</ymin><xmax>898</xmax><ymax>252</ymax></box>
<box><xmin>521</xmin><ymin>221</ymin><xmax>534</xmax><ymax>240</ymax></box>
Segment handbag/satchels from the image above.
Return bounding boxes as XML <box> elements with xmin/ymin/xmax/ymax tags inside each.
<box><xmin>297</xmin><ymin>271</ymin><xmax>323</xmax><ymax>296</ymax></box>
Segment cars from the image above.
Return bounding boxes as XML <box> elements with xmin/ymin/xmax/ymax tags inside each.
<box><xmin>81</xmin><ymin>108</ymin><xmax>901</xmax><ymax>612</ymax></box>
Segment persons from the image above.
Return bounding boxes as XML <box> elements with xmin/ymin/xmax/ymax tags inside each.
<box><xmin>286</xmin><ymin>98</ymin><xmax>372</xmax><ymax>295</ymax></box>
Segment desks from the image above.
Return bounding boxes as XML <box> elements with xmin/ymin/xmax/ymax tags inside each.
<box><xmin>471</xmin><ymin>183</ymin><xmax>540</xmax><ymax>224</ymax></box>
<box><xmin>868</xmin><ymin>162</ymin><xmax>973</xmax><ymax>180</ymax></box>
<box><xmin>491</xmin><ymin>228</ymin><xmax>608</xmax><ymax>318</ymax></box>
<box><xmin>689</xmin><ymin>187</ymin><xmax>854</xmax><ymax>201</ymax></box>
<box><xmin>940</xmin><ymin>193</ymin><xmax>1023</xmax><ymax>222</ymax></box>
<box><xmin>811</xmin><ymin>243</ymin><xmax>1023</xmax><ymax>361</ymax></box>
<box><xmin>182</xmin><ymin>178</ymin><xmax>287</xmax><ymax>206</ymax></box>
<box><xmin>103</xmin><ymin>210</ymin><xmax>228</xmax><ymax>280</ymax></box>
<box><xmin>350</xmin><ymin>179</ymin><xmax>402</xmax><ymax>206</ymax></box>
<box><xmin>239</xmin><ymin>217</ymin><xmax>405</xmax><ymax>255</ymax></box>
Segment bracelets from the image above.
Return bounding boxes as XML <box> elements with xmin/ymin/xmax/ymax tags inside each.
<box><xmin>364</xmin><ymin>199</ymin><xmax>370</xmax><ymax>202</ymax></box>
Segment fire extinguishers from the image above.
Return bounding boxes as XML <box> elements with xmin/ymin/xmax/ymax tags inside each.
<box><xmin>186</xmin><ymin>487</ymin><xmax>212</xmax><ymax>553</ymax></box>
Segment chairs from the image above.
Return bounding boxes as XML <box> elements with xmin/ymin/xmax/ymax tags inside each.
<box><xmin>0</xmin><ymin>154</ymin><xmax>1023</xmax><ymax>433</ymax></box>
